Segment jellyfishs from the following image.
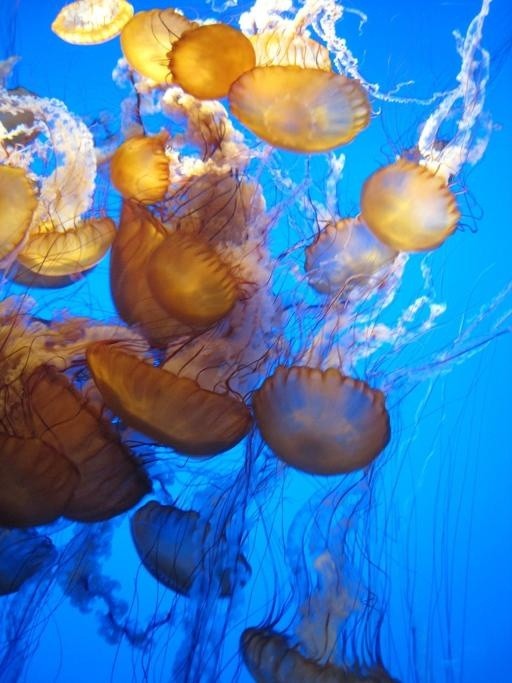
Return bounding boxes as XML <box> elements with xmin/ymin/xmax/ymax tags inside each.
<box><xmin>0</xmin><ymin>0</ymin><xmax>511</xmax><ymax>683</ymax></box>
